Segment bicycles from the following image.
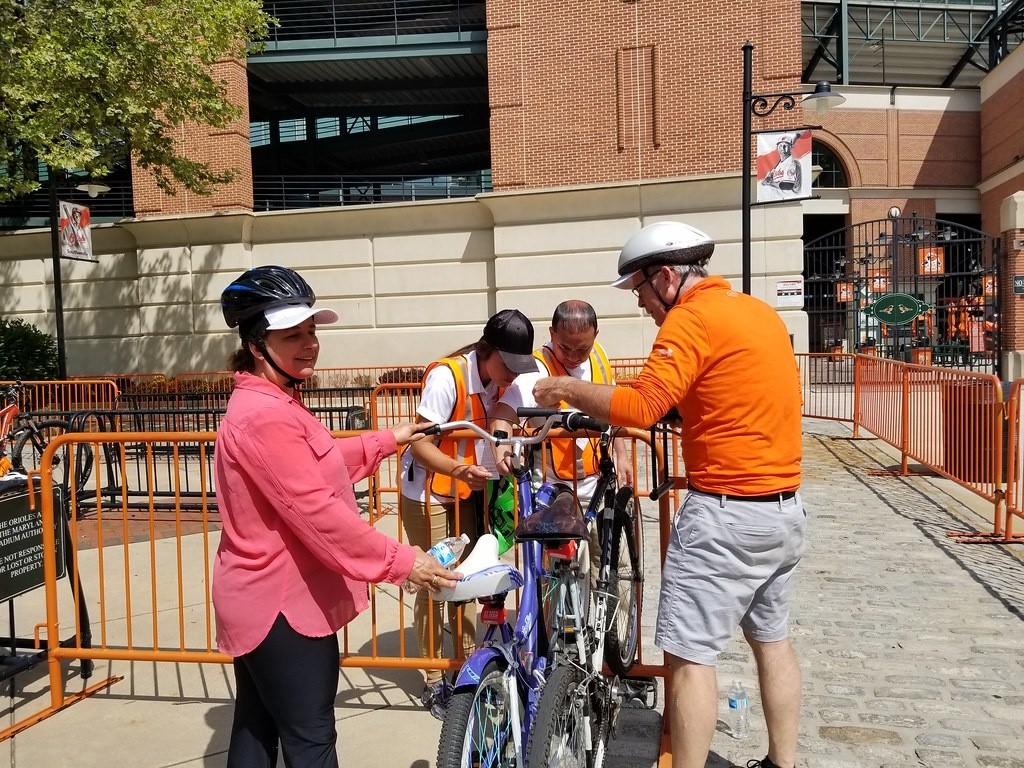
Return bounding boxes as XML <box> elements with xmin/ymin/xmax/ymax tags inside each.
<box><xmin>405</xmin><ymin>407</ymin><xmax>649</xmax><ymax>768</ymax></box>
<box><xmin>0</xmin><ymin>379</ymin><xmax>93</xmax><ymax>504</ymax></box>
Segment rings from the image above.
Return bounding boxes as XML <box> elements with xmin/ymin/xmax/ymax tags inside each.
<box><xmin>432</xmin><ymin>576</ymin><xmax>437</xmax><ymax>584</ymax></box>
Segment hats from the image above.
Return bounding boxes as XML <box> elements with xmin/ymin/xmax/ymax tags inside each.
<box><xmin>264</xmin><ymin>303</ymin><xmax>339</xmax><ymax>330</ymax></box>
<box><xmin>610</xmin><ymin>269</ymin><xmax>641</xmax><ymax>290</ymax></box>
<box><xmin>776</xmin><ymin>136</ymin><xmax>792</xmax><ymax>145</ymax></box>
<box><xmin>484</xmin><ymin>309</ymin><xmax>540</xmax><ymax>373</ymax></box>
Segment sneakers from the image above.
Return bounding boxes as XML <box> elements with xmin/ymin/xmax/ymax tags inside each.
<box><xmin>421</xmin><ymin>676</ymin><xmax>455</xmax><ymax>720</ymax></box>
<box><xmin>451</xmin><ymin>670</ymin><xmax>501</xmax><ymax>705</ymax></box>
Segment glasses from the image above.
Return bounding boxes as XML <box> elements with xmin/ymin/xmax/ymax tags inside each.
<box><xmin>632</xmin><ymin>267</ymin><xmax>676</xmax><ymax>298</ymax></box>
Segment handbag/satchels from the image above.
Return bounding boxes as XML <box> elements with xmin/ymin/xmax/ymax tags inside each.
<box><xmin>487</xmin><ymin>473</ymin><xmax>517</xmax><ymax>556</ymax></box>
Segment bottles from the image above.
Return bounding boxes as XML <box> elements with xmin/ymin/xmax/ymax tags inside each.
<box><xmin>728</xmin><ymin>679</ymin><xmax>750</xmax><ymax>739</ymax></box>
<box><xmin>399</xmin><ymin>533</ymin><xmax>471</xmax><ymax>595</ymax></box>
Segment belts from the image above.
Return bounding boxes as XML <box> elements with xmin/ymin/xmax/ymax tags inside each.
<box><xmin>687</xmin><ymin>478</ymin><xmax>795</xmax><ymax>502</ymax></box>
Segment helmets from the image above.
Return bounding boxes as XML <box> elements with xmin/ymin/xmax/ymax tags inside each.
<box><xmin>618</xmin><ymin>221</ymin><xmax>714</xmax><ymax>276</ymax></box>
<box><xmin>220</xmin><ymin>265</ymin><xmax>316</xmax><ymax>328</ymax></box>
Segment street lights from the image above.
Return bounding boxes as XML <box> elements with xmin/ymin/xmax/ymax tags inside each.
<box><xmin>741</xmin><ymin>42</ymin><xmax>846</xmax><ymax>300</ymax></box>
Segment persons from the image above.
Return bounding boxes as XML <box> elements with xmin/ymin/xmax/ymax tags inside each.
<box><xmin>395</xmin><ymin>310</ymin><xmax>540</xmax><ymax>722</ymax></box>
<box><xmin>61</xmin><ymin>207</ymin><xmax>90</xmax><ymax>259</ymax></box>
<box><xmin>211</xmin><ymin>266</ymin><xmax>462</xmax><ymax>767</ymax></box>
<box><xmin>533</xmin><ymin>221</ymin><xmax>809</xmax><ymax>766</ymax></box>
<box><xmin>488</xmin><ymin>301</ymin><xmax>634</xmax><ymax>642</ymax></box>
<box><xmin>766</xmin><ymin>136</ymin><xmax>801</xmax><ymax>198</ymax></box>
